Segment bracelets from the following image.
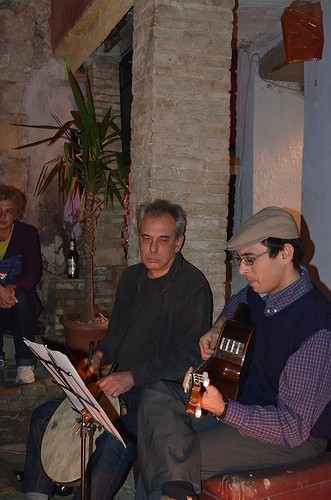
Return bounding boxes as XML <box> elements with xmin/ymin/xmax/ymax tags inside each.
<box><xmin>213</xmin><ymin>398</ymin><xmax>230</xmax><ymax>421</ymax></box>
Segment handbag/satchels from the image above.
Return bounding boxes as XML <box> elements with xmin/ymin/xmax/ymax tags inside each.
<box><xmin>0</xmin><ymin>255</ymin><xmax>23</xmax><ymax>286</ymax></box>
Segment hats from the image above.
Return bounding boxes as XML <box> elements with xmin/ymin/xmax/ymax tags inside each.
<box><xmin>223</xmin><ymin>206</ymin><xmax>299</xmax><ymax>251</ymax></box>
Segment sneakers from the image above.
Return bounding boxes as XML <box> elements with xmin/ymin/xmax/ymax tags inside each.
<box><xmin>15</xmin><ymin>365</ymin><xmax>36</xmax><ymax>385</ymax></box>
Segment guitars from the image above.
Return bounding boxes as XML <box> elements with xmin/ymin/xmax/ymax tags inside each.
<box><xmin>186</xmin><ymin>302</ymin><xmax>255</xmax><ymax>418</ymax></box>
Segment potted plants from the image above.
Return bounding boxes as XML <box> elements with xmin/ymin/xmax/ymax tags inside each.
<box><xmin>9</xmin><ymin>63</ymin><xmax>131</xmax><ymax>361</ymax></box>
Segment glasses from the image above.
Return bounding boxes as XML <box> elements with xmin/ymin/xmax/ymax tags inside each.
<box><xmin>235</xmin><ymin>249</ymin><xmax>270</xmax><ymax>266</ymax></box>
<box><xmin>0</xmin><ymin>208</ymin><xmax>14</xmax><ymax>215</ymax></box>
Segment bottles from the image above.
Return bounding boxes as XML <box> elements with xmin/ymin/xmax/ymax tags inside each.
<box><xmin>68</xmin><ymin>239</ymin><xmax>79</xmax><ymax>279</ymax></box>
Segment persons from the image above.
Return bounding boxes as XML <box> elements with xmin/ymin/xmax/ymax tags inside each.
<box><xmin>136</xmin><ymin>206</ymin><xmax>331</xmax><ymax>500</ymax></box>
<box><xmin>20</xmin><ymin>200</ymin><xmax>213</xmax><ymax>500</ymax></box>
<box><xmin>0</xmin><ymin>185</ymin><xmax>44</xmax><ymax>386</ymax></box>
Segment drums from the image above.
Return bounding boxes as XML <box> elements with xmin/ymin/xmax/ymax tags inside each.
<box><xmin>40</xmin><ymin>383</ymin><xmax>129</xmax><ymax>489</ymax></box>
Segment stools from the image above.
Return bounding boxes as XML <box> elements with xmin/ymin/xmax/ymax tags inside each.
<box><xmin>201</xmin><ymin>456</ymin><xmax>331</xmax><ymax>500</ymax></box>
